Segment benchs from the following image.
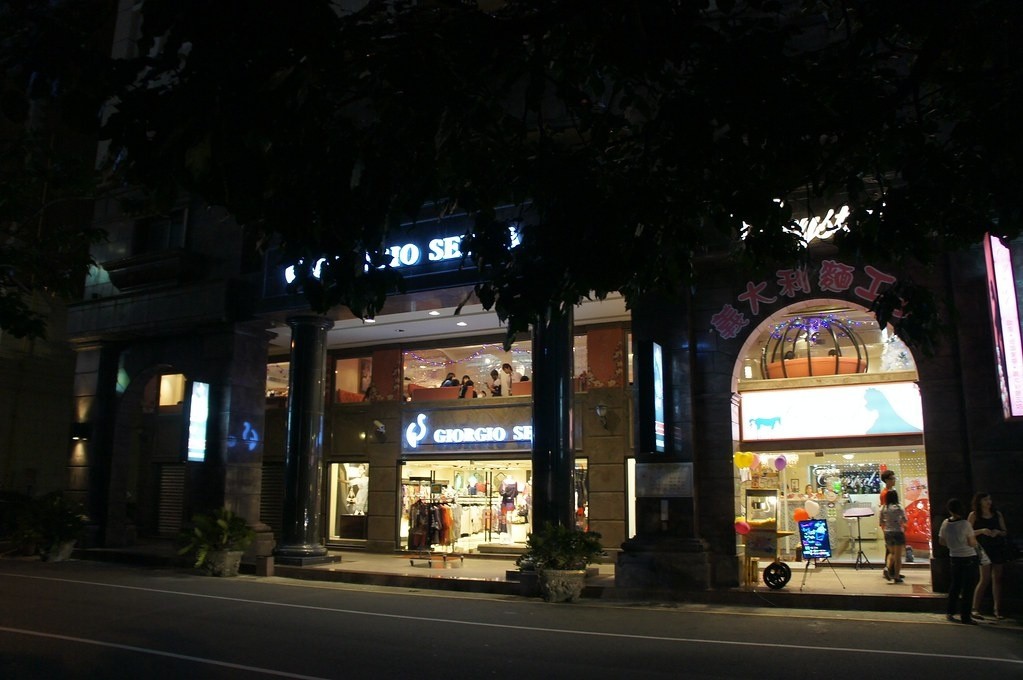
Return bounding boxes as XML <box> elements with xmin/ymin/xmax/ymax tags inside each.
<box><xmin>511</xmin><ymin>381</ymin><xmax>532</xmax><ymax>396</ymax></box>
<box><xmin>408</xmin><ymin>384</ymin><xmax>474</xmax><ymax>400</ymax></box>
<box><xmin>337</xmin><ymin>388</ymin><xmax>365</xmax><ymax>403</ymax></box>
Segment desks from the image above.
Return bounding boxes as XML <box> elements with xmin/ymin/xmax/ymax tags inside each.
<box><xmin>844</xmin><ymin>513</ymin><xmax>874</xmax><ymax>569</ymax></box>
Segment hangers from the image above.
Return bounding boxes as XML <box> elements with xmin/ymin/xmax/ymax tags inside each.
<box><xmin>417</xmin><ymin>497</ymin><xmax>457</xmax><ymax>509</ymax></box>
<box><xmin>460</xmin><ymin>502</ymin><xmax>486</xmax><ymax>507</ymax></box>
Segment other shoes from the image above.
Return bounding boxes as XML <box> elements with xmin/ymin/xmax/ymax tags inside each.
<box><xmin>889</xmin><ymin>573</ymin><xmax>905</xmax><ymax>579</ymax></box>
<box><xmin>884</xmin><ymin>567</ymin><xmax>891</xmax><ymax>581</ymax></box>
<box><xmin>894</xmin><ymin>578</ymin><xmax>903</xmax><ymax>583</ymax></box>
<box><xmin>970</xmin><ymin>610</ymin><xmax>985</xmax><ymax>621</ymax></box>
<box><xmin>993</xmin><ymin>612</ymin><xmax>1005</xmax><ymax>620</ymax></box>
<box><xmin>947</xmin><ymin>615</ymin><xmax>960</xmax><ymax>623</ymax></box>
<box><xmin>961</xmin><ymin>619</ymin><xmax>977</xmax><ymax>625</ymax></box>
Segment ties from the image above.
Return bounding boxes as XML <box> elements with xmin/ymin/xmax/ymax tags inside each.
<box><xmin>509</xmin><ymin>373</ymin><xmax>512</xmax><ymax>388</ymax></box>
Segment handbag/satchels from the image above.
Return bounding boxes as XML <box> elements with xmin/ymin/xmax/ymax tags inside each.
<box><xmin>973</xmin><ymin>541</ymin><xmax>991</xmax><ymax>566</ymax></box>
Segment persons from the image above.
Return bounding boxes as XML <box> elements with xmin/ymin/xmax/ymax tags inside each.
<box><xmin>441</xmin><ymin>373</ymin><xmax>478</xmax><ymax>398</ymax></box>
<box><xmin>784</xmin><ymin>351</ymin><xmax>795</xmax><ymax>361</ymax></box>
<box><xmin>968</xmin><ymin>490</ymin><xmax>1007</xmax><ymax>620</ymax></box>
<box><xmin>337</xmin><ymin>464</ymin><xmax>369</xmax><ymax>515</ymax></box>
<box><xmin>475</xmin><ymin>388</ymin><xmax>486</xmax><ymax>398</ymax></box>
<box><xmin>498</xmin><ymin>475</ymin><xmax>518</xmax><ymax>533</ymax></box>
<box><xmin>806</xmin><ymin>484</ymin><xmax>813</xmax><ymax>495</ymax></box>
<box><xmin>877</xmin><ymin>490</ymin><xmax>908</xmax><ymax>584</ymax></box>
<box><xmin>502</xmin><ymin>363</ymin><xmax>529</xmax><ymax>396</ymax></box>
<box><xmin>879</xmin><ymin>470</ymin><xmax>905</xmax><ymax>578</ymax></box>
<box><xmin>938</xmin><ymin>499</ymin><xmax>978</xmax><ymax>625</ymax></box>
<box><xmin>487</xmin><ymin>370</ymin><xmax>502</xmax><ymax>396</ymax></box>
<box><xmin>828</xmin><ymin>349</ymin><xmax>835</xmax><ymax>357</ymax></box>
<box><xmin>522</xmin><ymin>477</ymin><xmax>532</xmax><ymax>498</ymax></box>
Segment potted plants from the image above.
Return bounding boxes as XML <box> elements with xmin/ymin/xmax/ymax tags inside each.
<box><xmin>9</xmin><ymin>488</ymin><xmax>90</xmax><ymax>563</ymax></box>
<box><xmin>175</xmin><ymin>508</ymin><xmax>258</xmax><ymax>578</ymax></box>
<box><xmin>515</xmin><ymin>520</ymin><xmax>610</xmax><ymax>603</ymax></box>
